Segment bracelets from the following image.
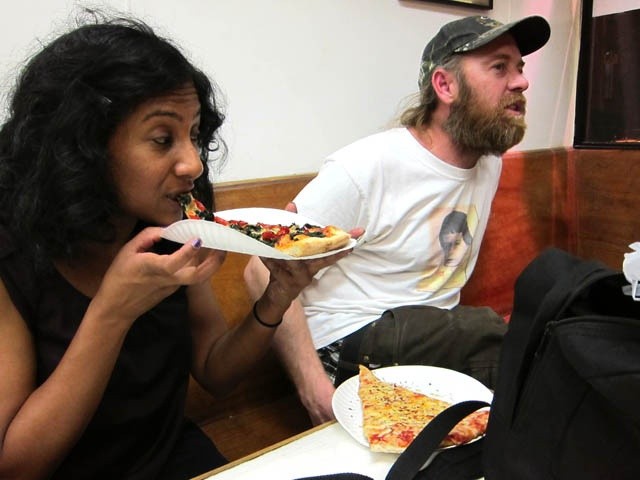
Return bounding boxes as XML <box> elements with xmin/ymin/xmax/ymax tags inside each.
<box><xmin>253</xmin><ymin>301</ymin><xmax>283</xmax><ymax>328</ymax></box>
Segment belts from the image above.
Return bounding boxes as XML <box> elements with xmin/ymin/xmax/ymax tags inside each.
<box><xmin>334</xmin><ymin>327</ymin><xmax>367</xmax><ymax>390</ymax></box>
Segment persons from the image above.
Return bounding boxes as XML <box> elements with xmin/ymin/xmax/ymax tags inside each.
<box><xmin>244</xmin><ymin>14</ymin><xmax>552</xmax><ymax>429</ymax></box>
<box><xmin>440</xmin><ymin>210</ymin><xmax>473</xmax><ymax>267</ymax></box>
<box><xmin>1</xmin><ymin>14</ymin><xmax>365</xmax><ymax>479</ymax></box>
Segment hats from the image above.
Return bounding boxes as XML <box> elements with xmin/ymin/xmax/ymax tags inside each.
<box><xmin>418</xmin><ymin>15</ymin><xmax>550</xmax><ymax>89</ymax></box>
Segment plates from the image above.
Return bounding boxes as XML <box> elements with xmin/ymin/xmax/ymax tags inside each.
<box><xmin>160</xmin><ymin>207</ymin><xmax>358</xmax><ymax>260</ymax></box>
<box><xmin>332</xmin><ymin>365</ymin><xmax>494</xmax><ymax>453</ymax></box>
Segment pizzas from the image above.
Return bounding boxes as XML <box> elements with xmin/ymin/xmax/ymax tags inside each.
<box><xmin>177</xmin><ymin>191</ymin><xmax>351</xmax><ymax>258</ymax></box>
<box><xmin>356</xmin><ymin>363</ymin><xmax>493</xmax><ymax>454</ymax></box>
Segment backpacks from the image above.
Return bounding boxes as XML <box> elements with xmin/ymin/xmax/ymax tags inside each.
<box><xmin>386</xmin><ymin>247</ymin><xmax>639</xmax><ymax>479</ymax></box>
